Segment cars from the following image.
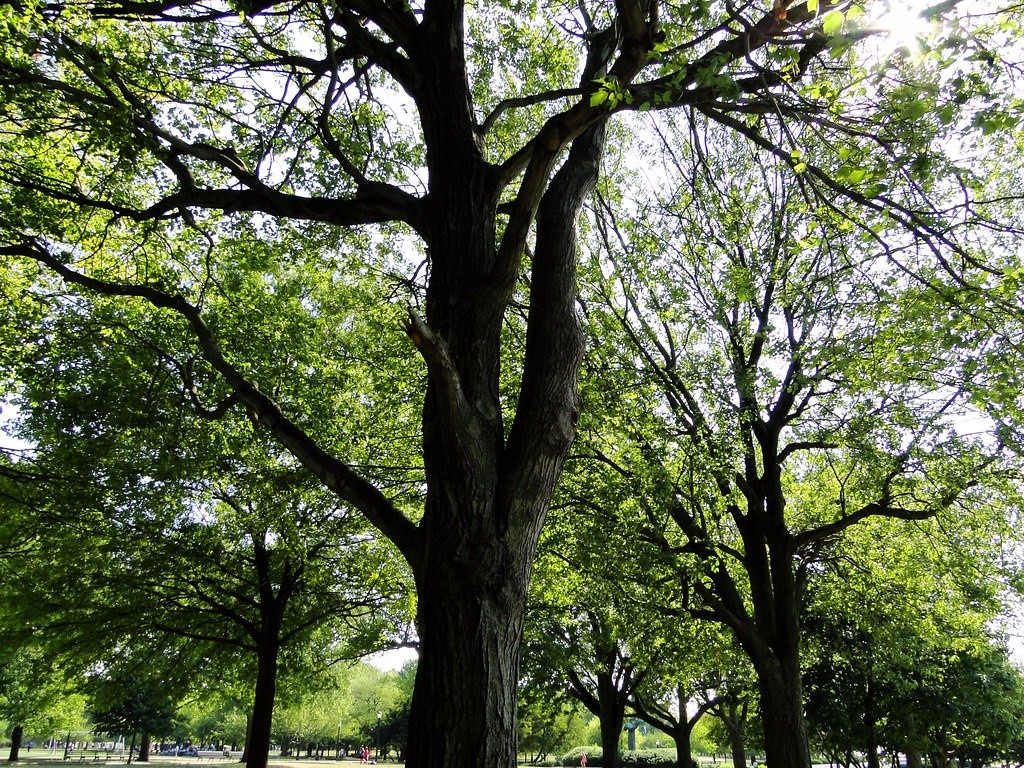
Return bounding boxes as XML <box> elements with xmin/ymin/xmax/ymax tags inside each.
<box><xmin>149</xmin><ymin>741</ymin><xmax>198</xmax><ymax>757</ymax></box>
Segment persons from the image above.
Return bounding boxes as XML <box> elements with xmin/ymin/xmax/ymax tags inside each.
<box><xmin>360</xmin><ymin>744</ymin><xmax>369</xmax><ymax>761</ymax></box>
<box><xmin>751</xmin><ymin>754</ymin><xmax>756</xmax><ymax>766</ymax></box>
<box><xmin>27</xmin><ymin>741</ymin><xmax>33</xmax><ymax>752</ymax></box>
<box><xmin>69</xmin><ymin>744</ymin><xmax>73</xmax><ymax>754</ymax></box>
